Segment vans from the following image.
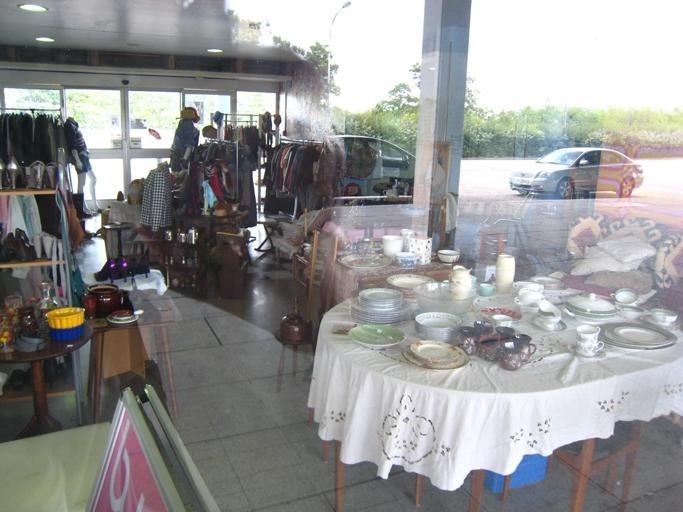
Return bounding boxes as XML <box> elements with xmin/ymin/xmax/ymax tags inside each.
<box><xmin>110</xmin><ymin>117</ymin><xmax>150</xmax><ymax>149</ymax></box>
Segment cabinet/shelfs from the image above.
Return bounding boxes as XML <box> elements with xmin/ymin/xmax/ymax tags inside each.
<box><xmin>0</xmin><ymin>186</ymin><xmax>82</xmax><ymax>405</ymax></box>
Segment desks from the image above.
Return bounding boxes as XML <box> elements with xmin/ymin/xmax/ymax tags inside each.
<box><xmin>84</xmin><ymin>284</ymin><xmax>182</xmax><ymax>429</ymax></box>
<box><xmin>0</xmin><ymin>302</ymin><xmax>95</xmax><ymax>442</ymax></box>
<box><xmin>100</xmin><ymin>206</ymin><xmax>252</xmax><ymax>301</ymax></box>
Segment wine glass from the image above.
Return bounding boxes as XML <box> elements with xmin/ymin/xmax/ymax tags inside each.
<box><xmin>0</xmin><ymin>325</ymin><xmax>14</xmax><ymax>353</ymax></box>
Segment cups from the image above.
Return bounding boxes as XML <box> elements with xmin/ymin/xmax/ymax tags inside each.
<box><xmin>618</xmin><ymin>285</ymin><xmax>678</xmax><ymax>322</ymax></box>
<box><xmin>399</xmin><ymin>229</ymin><xmax>414</xmax><ymax>251</ymax></box>
<box><xmin>471</xmin><ymin>323</ymin><xmax>538</xmax><ymax>371</ymax></box>
<box><xmin>5</xmin><ymin>295</ymin><xmax>21</xmax><ymax>312</ymax></box>
<box><xmin>357</xmin><ymin>243</ymin><xmax>372</xmax><ymax>265</ymax></box>
<box><xmin>479</xmin><ymin>283</ymin><xmax>493</xmax><ymax>295</ymax></box>
<box><xmin>568</xmin><ymin>324</ymin><xmax>603</xmax><ymax>353</ymax></box>
<box><xmin>494</xmin><ymin>254</ymin><xmax>515</xmax><ymax>293</ymax></box>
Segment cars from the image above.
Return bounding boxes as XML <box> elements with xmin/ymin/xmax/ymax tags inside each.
<box><xmin>508</xmin><ymin>147</ymin><xmax>644</xmax><ymax>199</ymax></box>
<box><xmin>328</xmin><ymin>133</ymin><xmax>415</xmax><ymax>205</ymax></box>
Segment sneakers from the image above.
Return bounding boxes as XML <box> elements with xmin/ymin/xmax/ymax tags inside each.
<box><xmin>0</xmin><ymin>228</ymin><xmax>55</xmax><ymax>262</ymax></box>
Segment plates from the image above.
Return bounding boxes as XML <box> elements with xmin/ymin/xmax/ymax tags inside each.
<box><xmin>350</xmin><ymin>323</ymin><xmax>408</xmax><ymax>350</ymax></box>
<box><xmin>385</xmin><ymin>275</ymin><xmax>437</xmax><ymax>298</ymax></box>
<box><xmin>481</xmin><ymin>307</ymin><xmax>522</xmax><ymax>325</ymax></box>
<box><xmin>564</xmin><ymin>291</ymin><xmax>618</xmax><ymax>319</ymax></box>
<box><xmin>337</xmin><ymin>253</ymin><xmax>394</xmax><ymax>270</ymax></box>
<box><xmin>415</xmin><ymin>312</ymin><xmax>462</xmax><ymax>345</ymax></box>
<box><xmin>600</xmin><ymin>322</ymin><xmax>677</xmax><ymax>351</ymax></box>
<box><xmin>402</xmin><ymin>346</ymin><xmax>470</xmax><ymax>369</ymax></box>
<box><xmin>107</xmin><ymin>310</ymin><xmax>138</xmax><ymax>325</ymax></box>
<box><xmin>410</xmin><ymin>340</ymin><xmax>463</xmax><ymax>365</ymax></box>
<box><xmin>531</xmin><ymin>275</ymin><xmax>564</xmax><ymax>291</ymax></box>
<box><xmin>532</xmin><ymin>314</ymin><xmax>567</xmax><ymax>333</ymax></box>
<box><xmin>349</xmin><ymin>288</ymin><xmax>410</xmax><ymax>325</ymax></box>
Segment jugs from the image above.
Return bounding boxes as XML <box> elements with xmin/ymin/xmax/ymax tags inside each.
<box><xmin>516</xmin><ymin>288</ymin><xmax>543</xmax><ymax>306</ymax></box>
<box><xmin>614</xmin><ymin>305</ymin><xmax>652</xmax><ymax>320</ymax></box>
<box><xmin>536</xmin><ymin>300</ymin><xmax>562</xmax><ymax>327</ymax></box>
<box><xmin>609</xmin><ymin>287</ymin><xmax>657</xmax><ymax>306</ymax></box>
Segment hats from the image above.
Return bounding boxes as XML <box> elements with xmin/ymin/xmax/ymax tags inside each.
<box><xmin>176</xmin><ymin>108</ymin><xmax>196</xmax><ymax>121</ymax></box>
<box><xmin>212</xmin><ymin>111</ymin><xmax>224</xmax><ymax>123</ymax></box>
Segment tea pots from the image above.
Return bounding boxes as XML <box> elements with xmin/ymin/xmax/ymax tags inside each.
<box><xmin>448</xmin><ymin>265</ymin><xmax>474</xmax><ymax>300</ymax></box>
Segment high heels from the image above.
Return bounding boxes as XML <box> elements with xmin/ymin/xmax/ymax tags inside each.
<box><xmin>0</xmin><ymin>157</ymin><xmax>58</xmax><ymax>189</ymax></box>
<box><xmin>130</xmin><ymin>257</ymin><xmax>137</xmax><ymax>281</ymax></box>
<box><xmin>0</xmin><ymin>360</ymin><xmax>70</xmax><ymax>395</ymax></box>
<box><xmin>95</xmin><ymin>259</ymin><xmax>116</xmax><ymax>284</ymax></box>
<box><xmin>118</xmin><ymin>257</ymin><xmax>129</xmax><ymax>282</ymax></box>
<box><xmin>138</xmin><ymin>255</ymin><xmax>149</xmax><ymax>278</ymax></box>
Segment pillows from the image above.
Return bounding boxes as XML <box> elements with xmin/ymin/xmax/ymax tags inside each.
<box><xmin>567</xmin><ymin>236</ymin><xmax>657</xmax><ymax>295</ymax></box>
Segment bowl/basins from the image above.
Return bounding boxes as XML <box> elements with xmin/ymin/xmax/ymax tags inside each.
<box><xmin>438</xmin><ymin>249</ymin><xmax>461</xmax><ymax>265</ymax></box>
<box><xmin>648</xmin><ymin>307</ymin><xmax>678</xmax><ymax>327</ymax></box>
<box><xmin>382</xmin><ymin>236</ymin><xmax>402</xmax><ymax>256</ymax></box>
<box><xmin>394</xmin><ymin>251</ymin><xmax>419</xmax><ymax>269</ymax></box>
<box><xmin>46</xmin><ymin>305</ymin><xmax>85</xmax><ymax>341</ymax></box>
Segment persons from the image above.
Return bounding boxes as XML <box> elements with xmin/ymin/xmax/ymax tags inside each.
<box><xmin>64</xmin><ymin>116</ymin><xmax>106</xmax><ymax>217</ymax></box>
<box><xmin>139</xmin><ymin>161</ymin><xmax>174</xmax><ymax>233</ymax></box>
<box><xmin>167</xmin><ymin>107</ymin><xmax>200</xmax><ymax>181</ymax></box>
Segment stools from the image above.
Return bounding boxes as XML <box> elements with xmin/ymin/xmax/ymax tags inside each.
<box><xmin>271</xmin><ymin>332</ymin><xmax>316</xmax><ymax>395</ymax></box>
<box><xmin>254</xmin><ymin>216</ymin><xmax>279</xmax><ymax>251</ymax></box>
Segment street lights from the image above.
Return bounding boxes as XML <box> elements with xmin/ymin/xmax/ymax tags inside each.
<box><xmin>326</xmin><ymin>0</ymin><xmax>353</xmax><ymax>132</ymax></box>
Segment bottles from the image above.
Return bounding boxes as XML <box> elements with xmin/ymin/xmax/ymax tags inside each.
<box><xmin>164</xmin><ymin>227</ymin><xmax>198</xmax><ymax>267</ymax></box>
<box><xmin>19</xmin><ymin>313</ymin><xmax>35</xmax><ymax>338</ymax></box>
<box><xmin>34</xmin><ymin>282</ymin><xmax>56</xmax><ymax>331</ymax></box>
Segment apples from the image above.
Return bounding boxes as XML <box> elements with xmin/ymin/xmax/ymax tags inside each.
<box><xmin>82</xmin><ymin>295</ymin><xmax>97</xmax><ymax>312</ymax></box>
